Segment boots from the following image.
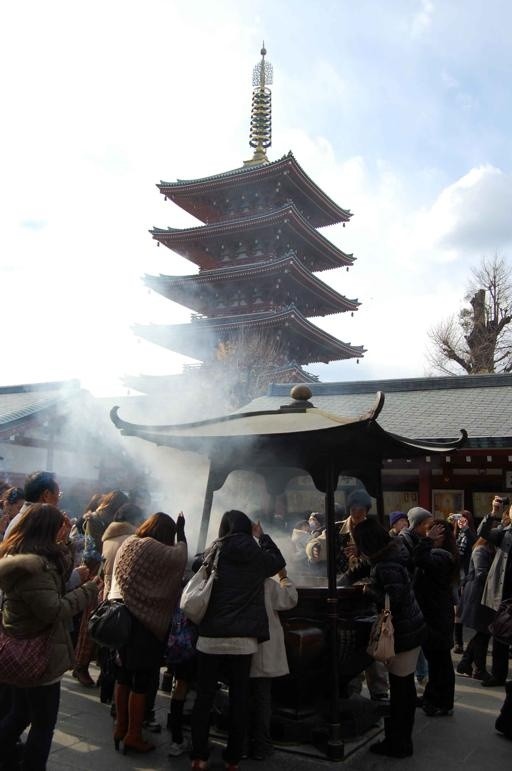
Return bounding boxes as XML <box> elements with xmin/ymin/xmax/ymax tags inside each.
<box><xmin>114</xmin><ymin>697</ymin><xmax>129</xmax><ymax>751</ymax></box>
<box><xmin>122</xmin><ymin>704</ymin><xmax>157</xmax><ymax>754</ymax></box>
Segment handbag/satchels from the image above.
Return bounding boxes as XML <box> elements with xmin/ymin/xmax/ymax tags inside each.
<box><xmin>167</xmin><ymin>592</ymin><xmax>199</xmax><ymax>662</ymax></box>
<box><xmin>487</xmin><ymin>607</ymin><xmax>512</xmax><ymax>646</ymax></box>
<box><xmin>0</xmin><ymin>620</ymin><xmax>57</xmax><ymax>686</ymax></box>
<box><xmin>85</xmin><ymin>589</ymin><xmax>136</xmax><ymax>650</ymax></box>
<box><xmin>366</xmin><ymin>592</ymin><xmax>397</xmax><ymax>663</ymax></box>
<box><xmin>179</xmin><ymin>542</ymin><xmax>221</xmax><ymax>627</ymax></box>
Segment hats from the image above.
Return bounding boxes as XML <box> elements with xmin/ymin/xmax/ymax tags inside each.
<box><xmin>310</xmin><ymin>511</ymin><xmax>324</xmax><ymax>526</ymax></box>
<box><xmin>406</xmin><ymin>506</ymin><xmax>431</xmax><ymax>532</ymax></box>
<box><xmin>389</xmin><ymin>510</ymin><xmax>407</xmax><ymax>529</ymax></box>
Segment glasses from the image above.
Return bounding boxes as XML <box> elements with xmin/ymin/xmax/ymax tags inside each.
<box><xmin>48</xmin><ymin>491</ymin><xmax>64</xmax><ymax>501</ymax></box>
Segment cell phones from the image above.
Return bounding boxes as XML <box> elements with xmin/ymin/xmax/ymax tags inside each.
<box><xmin>96</xmin><ymin>560</ymin><xmax>104</xmax><ymax>579</ymax></box>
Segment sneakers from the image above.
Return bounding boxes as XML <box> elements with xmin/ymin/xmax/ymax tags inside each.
<box><xmin>416</xmin><ymin>673</ymin><xmax>424</xmax><ymax>684</ymax></box>
<box><xmin>453</xmin><ymin>645</ymin><xmax>464</xmax><ymax>654</ymax></box>
<box><xmin>368</xmin><ymin>739</ymin><xmax>414</xmax><ymax>759</ymax></box>
<box><xmin>455</xmin><ymin>670</ymin><xmax>470</xmax><ymax>678</ymax></box>
<box><xmin>143</xmin><ymin>720</ymin><xmax>162</xmax><ymax>733</ymax></box>
<box><xmin>415</xmin><ymin>695</ymin><xmax>455</xmax><ymax>717</ymax></box>
<box><xmin>168</xmin><ymin>739</ymin><xmax>190</xmax><ymax>758</ymax></box>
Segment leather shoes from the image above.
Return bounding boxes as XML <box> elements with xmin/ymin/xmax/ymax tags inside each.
<box><xmin>241</xmin><ymin>754</ymin><xmax>249</xmax><ymax>760</ymax></box>
<box><xmin>71</xmin><ymin>666</ymin><xmax>95</xmax><ymax>688</ymax></box>
<box><xmin>222</xmin><ymin>761</ymin><xmax>237</xmax><ymax>771</ymax></box>
<box><xmin>254</xmin><ymin>754</ymin><xmax>265</xmax><ymax>762</ymax></box>
<box><xmin>189</xmin><ymin>760</ymin><xmax>208</xmax><ymax>771</ymax></box>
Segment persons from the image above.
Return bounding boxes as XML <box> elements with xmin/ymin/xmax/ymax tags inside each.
<box><xmin>388</xmin><ymin>509</ymin><xmax>409</xmax><ymax>536</ymax></box>
<box><xmin>88</xmin><ymin>510</ymin><xmax>188</xmax><ymax>756</ymax></box>
<box><xmin>128</xmin><ymin>486</ymin><xmax>152</xmax><ymax>519</ymax></box>
<box><xmin>1</xmin><ymin>480</ymin><xmax>10</xmax><ymax>494</ymax></box>
<box><xmin>244</xmin><ymin>568</ymin><xmax>299</xmax><ymax>762</ymax></box>
<box><xmin>0</xmin><ymin>502</ymin><xmax>105</xmax><ymax>770</ymax></box>
<box><xmin>495</xmin><ymin>645</ymin><xmax>511</xmax><ymax>739</ymax></box>
<box><xmin>397</xmin><ymin>506</ymin><xmax>433</xmax><ymax>708</ymax></box>
<box><xmin>307</xmin><ymin>511</ymin><xmax>326</xmax><ymax>538</ymax></box>
<box><xmin>1</xmin><ymin>486</ymin><xmax>25</xmax><ymax>542</ymax></box>
<box><xmin>450</xmin><ymin>511</ymin><xmax>475</xmax><ymax>653</ymax></box>
<box><xmin>291</xmin><ymin>520</ymin><xmax>313</xmax><ymax>562</ymax></box>
<box><xmin>411</xmin><ymin>519</ymin><xmax>457</xmax><ymax>717</ymax></box>
<box><xmin>477</xmin><ymin>495</ymin><xmax>511</xmax><ymax>687</ymax></box>
<box><xmin>454</xmin><ymin>519</ymin><xmax>493</xmax><ymax>681</ymax></box>
<box><xmin>182</xmin><ymin>509</ymin><xmax>286</xmax><ymax>770</ymax></box>
<box><xmin>1</xmin><ymin>471</ymin><xmax>90</xmax><ymax>592</ymax></box>
<box><xmin>73</xmin><ymin>490</ymin><xmax>130</xmax><ymax>687</ymax></box>
<box><xmin>305</xmin><ymin>490</ymin><xmax>372</xmax><ymax>578</ymax></box>
<box><xmin>337</xmin><ymin>519</ymin><xmax>425</xmax><ymax>759</ymax></box>
<box><xmin>102</xmin><ymin>502</ymin><xmax>161</xmax><ymax>733</ymax></box>
<box><xmin>69</xmin><ymin>493</ymin><xmax>105</xmax><ymax>650</ymax></box>
<box><xmin>164</xmin><ymin>584</ymin><xmax>201</xmax><ymax>758</ymax></box>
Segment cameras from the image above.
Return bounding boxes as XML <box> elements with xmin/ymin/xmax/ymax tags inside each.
<box><xmin>453</xmin><ymin>513</ymin><xmax>463</xmax><ymax>519</ymax></box>
<box><xmin>498</xmin><ymin>495</ymin><xmax>511</xmax><ymax>506</ymax></box>
